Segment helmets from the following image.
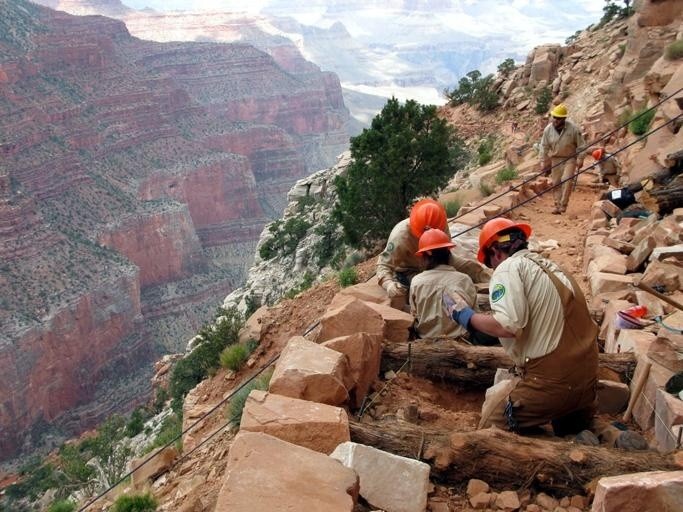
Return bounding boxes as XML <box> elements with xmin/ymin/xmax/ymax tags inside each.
<box><xmin>415</xmin><ymin>228</ymin><xmax>456</xmax><ymax>257</ymax></box>
<box><xmin>409</xmin><ymin>200</ymin><xmax>447</xmax><ymax>238</ymax></box>
<box><xmin>477</xmin><ymin>217</ymin><xmax>532</xmax><ymax>264</ymax></box>
<box><xmin>592</xmin><ymin>149</ymin><xmax>602</xmax><ymax>160</ymax></box>
<box><xmin>551</xmin><ymin>104</ymin><xmax>568</xmax><ymax>118</ymax></box>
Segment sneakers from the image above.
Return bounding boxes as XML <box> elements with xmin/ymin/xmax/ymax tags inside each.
<box><xmin>552</xmin><ymin>206</ymin><xmax>566</xmax><ymax>214</ymax></box>
<box><xmin>615</xmin><ymin>430</ymin><xmax>648</xmax><ymax>451</ymax></box>
<box><xmin>576</xmin><ymin>430</ymin><xmax>600</xmax><ymax>446</ymax></box>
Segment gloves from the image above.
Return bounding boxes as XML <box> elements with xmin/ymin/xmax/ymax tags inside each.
<box><xmin>381</xmin><ymin>280</ymin><xmax>409</xmax><ymax>297</ymax></box>
<box><xmin>443</xmin><ymin>287</ymin><xmax>475</xmax><ymax>334</ymax></box>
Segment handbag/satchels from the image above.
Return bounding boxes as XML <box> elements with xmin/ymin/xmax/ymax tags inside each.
<box><xmin>607</xmin><ymin>188</ymin><xmax>635</xmax><ymax>210</ymax></box>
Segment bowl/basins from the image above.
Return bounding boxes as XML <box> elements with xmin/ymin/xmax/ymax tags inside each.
<box><xmin>616</xmin><ymin>310</ymin><xmax>643</xmax><ymax>332</ymax></box>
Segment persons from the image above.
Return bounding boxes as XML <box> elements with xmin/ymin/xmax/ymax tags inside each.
<box><xmin>375</xmin><ymin>199</ymin><xmax>492</xmax><ymax>299</ymax></box>
<box><xmin>408</xmin><ymin>229</ymin><xmax>480</xmax><ymax>343</ymax></box>
<box><xmin>591</xmin><ymin>148</ymin><xmax>623</xmax><ymax>187</ymax></box>
<box><xmin>442</xmin><ymin>218</ymin><xmax>650</xmax><ymax>451</ymax></box>
<box><xmin>538</xmin><ymin>104</ymin><xmax>587</xmax><ymax>215</ymax></box>
